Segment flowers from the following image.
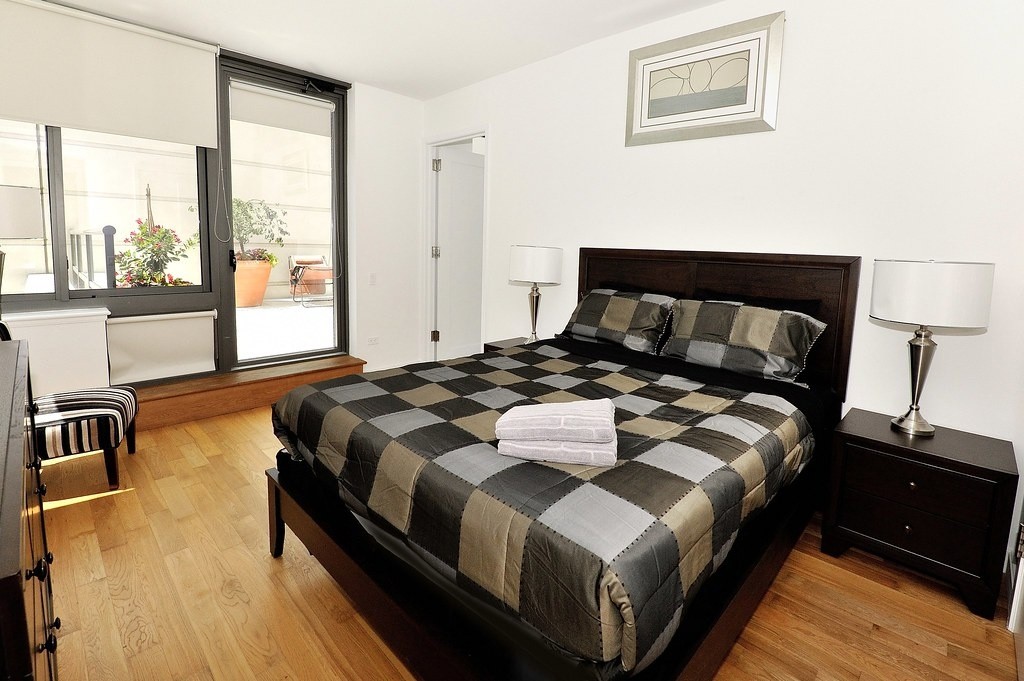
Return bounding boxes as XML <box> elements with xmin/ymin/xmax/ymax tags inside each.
<box><xmin>109</xmin><ymin>220</ymin><xmax>193</xmax><ymax>287</ymax></box>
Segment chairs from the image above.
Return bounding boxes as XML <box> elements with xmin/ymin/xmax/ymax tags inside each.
<box><xmin>0</xmin><ymin>322</ymin><xmax>139</xmax><ymax>492</ymax></box>
<box><xmin>288</xmin><ymin>254</ymin><xmax>334</xmax><ymax>307</ymax></box>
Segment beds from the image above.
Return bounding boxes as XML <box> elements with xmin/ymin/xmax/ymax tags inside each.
<box><xmin>264</xmin><ymin>248</ymin><xmax>863</xmax><ymax>681</ymax></box>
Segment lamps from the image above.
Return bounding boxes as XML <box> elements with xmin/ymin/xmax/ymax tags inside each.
<box><xmin>508</xmin><ymin>244</ymin><xmax>563</xmax><ymax>345</ymax></box>
<box><xmin>867</xmin><ymin>258</ymin><xmax>996</xmax><ymax>438</ymax></box>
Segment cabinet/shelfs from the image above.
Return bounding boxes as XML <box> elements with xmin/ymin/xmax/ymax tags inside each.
<box><xmin>0</xmin><ymin>339</ymin><xmax>61</xmax><ymax>681</ymax></box>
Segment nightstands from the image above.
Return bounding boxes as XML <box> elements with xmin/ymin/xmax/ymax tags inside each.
<box><xmin>819</xmin><ymin>409</ymin><xmax>1019</xmax><ymax>621</ymax></box>
<box><xmin>483</xmin><ymin>337</ymin><xmax>529</xmax><ymax>354</ymax></box>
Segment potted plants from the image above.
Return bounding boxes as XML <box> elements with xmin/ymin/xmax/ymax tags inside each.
<box><xmin>187</xmin><ymin>196</ymin><xmax>292</xmax><ymax>308</ymax></box>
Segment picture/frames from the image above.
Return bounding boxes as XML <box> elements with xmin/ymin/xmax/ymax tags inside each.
<box><xmin>624</xmin><ymin>10</ymin><xmax>785</xmax><ymax>147</ymax></box>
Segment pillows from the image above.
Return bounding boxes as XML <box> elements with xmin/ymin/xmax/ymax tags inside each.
<box><xmin>553</xmin><ymin>289</ymin><xmax>676</xmax><ymax>356</ymax></box>
<box><xmin>658</xmin><ymin>299</ymin><xmax>827</xmax><ymax>390</ymax></box>
